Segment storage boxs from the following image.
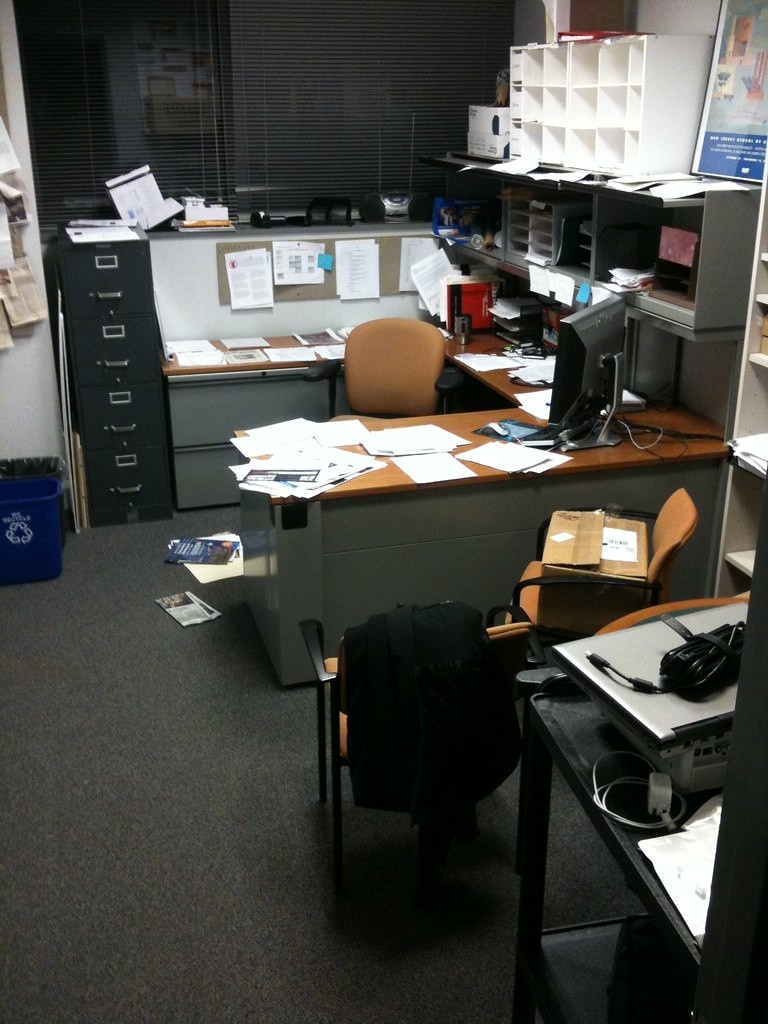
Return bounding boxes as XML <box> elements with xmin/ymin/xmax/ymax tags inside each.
<box><xmin>104</xmin><ymin>165</ymin><xmax>163</xmax><ymax>220</ymax></box>
<box><xmin>182</xmin><ymin>204</ymin><xmax>228</xmax><ymax>220</ymax></box>
<box><xmin>467</xmin><ymin>106</ymin><xmax>510</xmax><ymax>159</ymax></box>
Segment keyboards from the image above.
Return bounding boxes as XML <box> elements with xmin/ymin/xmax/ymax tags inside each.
<box><xmin>524</xmin><ymin>418</ymin><xmax>601</xmax><ymax>447</ymax></box>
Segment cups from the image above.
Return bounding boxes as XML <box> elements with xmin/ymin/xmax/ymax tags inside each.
<box><xmin>453</xmin><ymin>314</ymin><xmax>472</xmax><ymax>346</ymax></box>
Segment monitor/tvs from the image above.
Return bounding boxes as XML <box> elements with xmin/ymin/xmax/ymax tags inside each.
<box><xmin>549</xmin><ymin>294</ymin><xmax>628</xmax><ymax>452</ymax></box>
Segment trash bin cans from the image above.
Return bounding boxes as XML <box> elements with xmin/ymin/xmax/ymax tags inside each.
<box><xmin>1</xmin><ymin>455</ymin><xmax>64</xmax><ymax>585</ymax></box>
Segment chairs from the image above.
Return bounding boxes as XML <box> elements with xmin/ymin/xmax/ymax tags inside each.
<box><xmin>326</xmin><ymin>318</ymin><xmax>466</xmax><ymax>423</ymax></box>
<box><xmin>504</xmin><ymin>488</ymin><xmax>698</xmax><ymax>670</ymax></box>
<box><xmin>298</xmin><ymin>606</ymin><xmax>547</xmax><ymax>861</ymax></box>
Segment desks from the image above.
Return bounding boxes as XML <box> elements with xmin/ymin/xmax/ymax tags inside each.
<box><xmin>163</xmin><ymin>325</ymin><xmax>729</xmax><ymax>685</ymax></box>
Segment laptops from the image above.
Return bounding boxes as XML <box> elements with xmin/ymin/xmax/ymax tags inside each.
<box><xmin>552</xmin><ymin>601</ymin><xmax>748</xmax><ymax>760</ymax></box>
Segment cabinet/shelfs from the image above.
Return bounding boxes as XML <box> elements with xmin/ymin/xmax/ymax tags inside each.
<box><xmin>711</xmin><ymin>169</ymin><xmax>768</xmax><ymax>601</ymax></box>
<box><xmin>512</xmin><ymin>470</ymin><xmax>768</xmax><ymax>1024</ymax></box>
<box><xmin>510</xmin><ymin>34</ymin><xmax>715</xmax><ymax>176</ymax></box>
<box><xmin>506</xmin><ymin>199</ymin><xmax>591</xmax><ymax>266</ymax></box>
<box><xmin>59</xmin><ymin>224</ymin><xmax>174</xmax><ymax>527</ymax></box>
<box><xmin>424</xmin><ymin>156</ymin><xmax>763</xmax><ymax>341</ymax></box>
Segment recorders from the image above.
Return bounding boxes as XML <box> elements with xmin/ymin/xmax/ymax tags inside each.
<box><xmin>359</xmin><ymin>189</ymin><xmax>435</xmax><ymax>223</ymax></box>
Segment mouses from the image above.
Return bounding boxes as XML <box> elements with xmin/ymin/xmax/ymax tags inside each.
<box><xmin>480</xmin><ymin>422</ymin><xmax>511</xmax><ymax>436</ymax></box>
